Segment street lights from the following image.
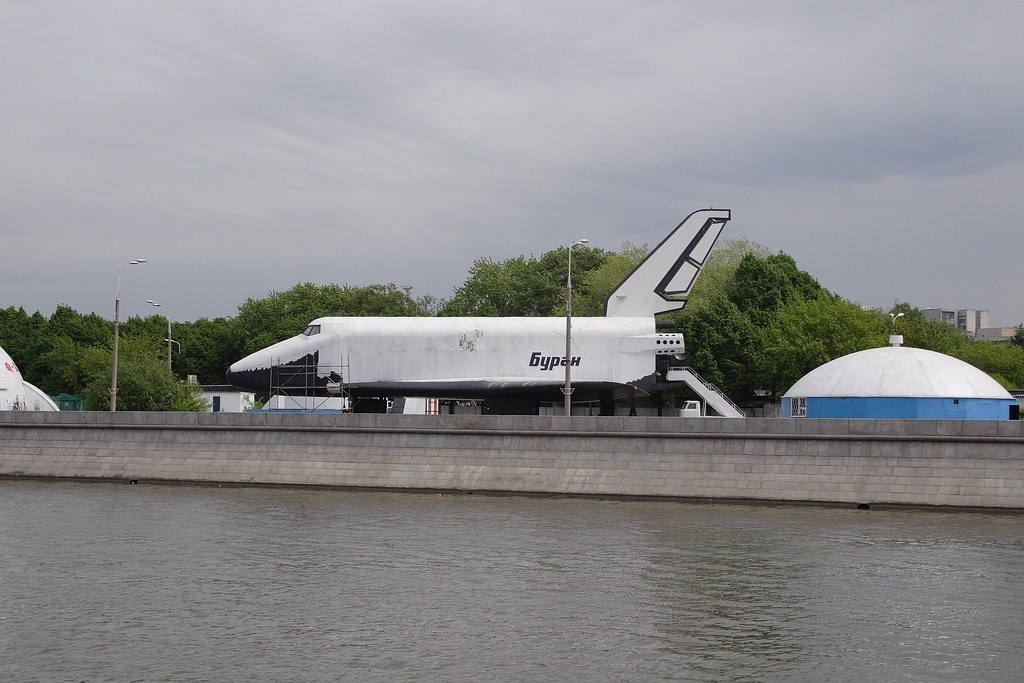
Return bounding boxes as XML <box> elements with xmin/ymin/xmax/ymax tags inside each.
<box><xmin>565</xmin><ymin>238</ymin><xmax>588</xmax><ymax>417</ymax></box>
<box><xmin>111</xmin><ymin>258</ymin><xmax>146</xmax><ymax>411</ymax></box>
<box><xmin>147</xmin><ymin>300</ymin><xmax>171</xmax><ymax>376</ymax></box>
<box><xmin>163</xmin><ymin>339</ymin><xmax>181</xmax><ymax>355</ymax></box>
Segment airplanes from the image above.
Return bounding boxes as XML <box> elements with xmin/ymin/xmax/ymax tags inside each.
<box><xmin>225</xmin><ymin>209</ymin><xmax>753</xmax><ymax>417</ymax></box>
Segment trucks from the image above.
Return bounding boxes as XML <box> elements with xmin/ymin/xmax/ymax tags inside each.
<box><xmin>678</xmin><ymin>399</ymin><xmax>708</xmax><ymax>417</ymax></box>
